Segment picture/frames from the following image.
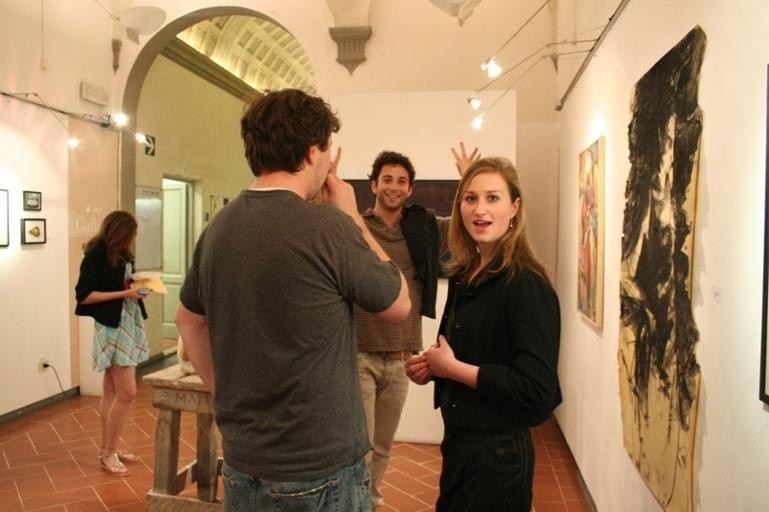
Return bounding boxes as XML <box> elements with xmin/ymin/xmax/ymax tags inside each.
<box><xmin>578</xmin><ymin>136</ymin><xmax>605</xmax><ymax>328</ymax></box>
<box><xmin>0</xmin><ymin>189</ymin><xmax>9</xmax><ymax>247</ymax></box>
<box><xmin>24</xmin><ymin>191</ymin><xmax>41</xmax><ymax>210</ymax></box>
<box><xmin>21</xmin><ymin>218</ymin><xmax>46</xmax><ymax>244</ymax></box>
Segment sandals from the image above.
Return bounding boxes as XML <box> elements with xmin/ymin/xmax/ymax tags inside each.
<box><xmin>98</xmin><ymin>453</ymin><xmax>132</xmax><ymax>477</ymax></box>
<box><xmin>118</xmin><ymin>449</ymin><xmax>137</xmax><ymax>463</ymax></box>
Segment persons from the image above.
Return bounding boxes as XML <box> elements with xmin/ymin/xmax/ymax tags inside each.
<box><xmin>72</xmin><ymin>207</ymin><xmax>156</xmax><ymax>476</ymax></box>
<box><xmin>330</xmin><ymin>138</ymin><xmax>484</xmax><ymax>512</ymax></box>
<box><xmin>403</xmin><ymin>155</ymin><xmax>563</xmax><ymax>512</ymax></box>
<box><xmin>170</xmin><ymin>87</ymin><xmax>412</xmax><ymax>512</ymax></box>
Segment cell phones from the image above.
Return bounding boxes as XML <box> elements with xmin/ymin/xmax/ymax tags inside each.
<box><xmin>138</xmin><ymin>288</ymin><xmax>150</xmax><ymax>296</ymax></box>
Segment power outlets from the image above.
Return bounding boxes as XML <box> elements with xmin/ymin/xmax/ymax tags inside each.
<box><xmin>39</xmin><ymin>360</ymin><xmax>49</xmax><ymax>372</ymax></box>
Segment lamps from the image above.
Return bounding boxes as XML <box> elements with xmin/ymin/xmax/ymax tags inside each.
<box><xmin>0</xmin><ymin>90</ymin><xmax>146</xmax><ymax>151</ymax></box>
<box><xmin>467</xmin><ymin>0</ymin><xmax>630</xmax><ymax>130</ymax></box>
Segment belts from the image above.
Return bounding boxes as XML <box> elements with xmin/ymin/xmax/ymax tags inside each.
<box><xmin>372</xmin><ymin>352</ymin><xmax>410</xmax><ymax>359</ymax></box>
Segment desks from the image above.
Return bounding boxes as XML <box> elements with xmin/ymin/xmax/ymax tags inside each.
<box><xmin>142</xmin><ymin>364</ymin><xmax>222</xmax><ymax>512</ymax></box>
